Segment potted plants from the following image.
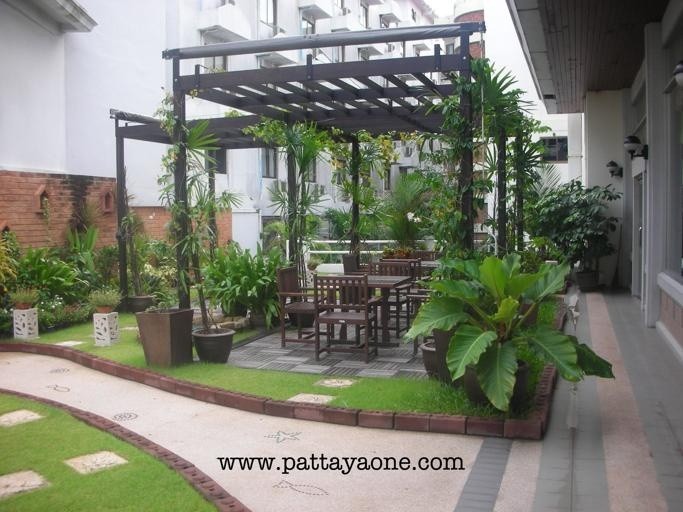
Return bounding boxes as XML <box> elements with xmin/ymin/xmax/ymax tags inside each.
<box><xmin>119</xmin><ymin>189</ymin><xmax>154</xmax><ymax>314</ymax></box>
<box><xmin>158</xmin><ymin>115</ymin><xmax>331</xmax><ymax>364</ymax></box>
<box><xmin>404</xmin><ymin>253</ymin><xmax>616</xmax><ymax>413</ymax></box>
<box><xmin>88</xmin><ymin>285</ymin><xmax>127</xmax><ymax>314</ymax></box>
<box><xmin>11</xmin><ymin>285</ymin><xmax>40</xmax><ymax>309</ymax></box>
<box><xmin>534</xmin><ymin>178</ymin><xmax>625</xmax><ymax>292</ymax></box>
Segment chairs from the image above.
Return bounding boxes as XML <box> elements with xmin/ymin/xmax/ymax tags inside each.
<box><xmin>278</xmin><ymin>257</ymin><xmax>433</xmax><ymax>363</ymax></box>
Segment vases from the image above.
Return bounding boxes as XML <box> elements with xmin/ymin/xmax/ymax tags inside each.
<box><xmin>136</xmin><ymin>308</ymin><xmax>193</xmax><ymax>368</ymax></box>
<box><xmin>420</xmin><ymin>340</ymin><xmax>435</xmax><ymax>378</ymax></box>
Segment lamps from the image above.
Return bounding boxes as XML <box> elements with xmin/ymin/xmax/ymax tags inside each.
<box><xmin>624</xmin><ymin>136</ymin><xmax>647</xmax><ymax>160</ymax></box>
<box><xmin>606</xmin><ymin>160</ymin><xmax>622</xmax><ymax>178</ymax></box>
